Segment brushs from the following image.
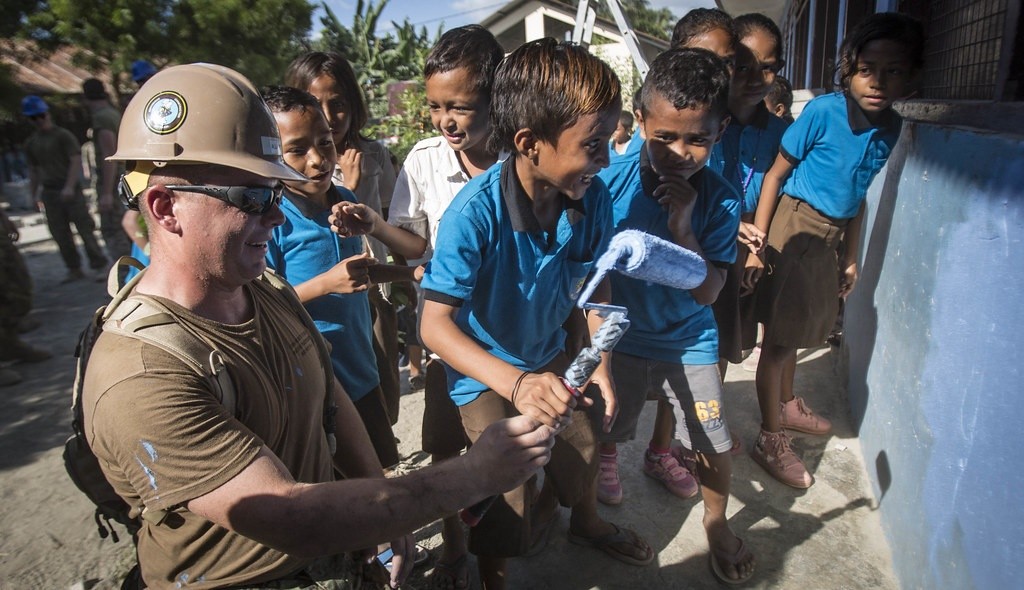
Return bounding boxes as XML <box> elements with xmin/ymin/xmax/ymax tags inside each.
<box><xmin>457</xmin><ymin>227</ymin><xmax>704</xmax><ymax>528</ymax></box>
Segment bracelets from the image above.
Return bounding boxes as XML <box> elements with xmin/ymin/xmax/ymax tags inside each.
<box><xmin>512</xmin><ymin>372</ymin><xmax>530</xmax><ymax>407</ymax></box>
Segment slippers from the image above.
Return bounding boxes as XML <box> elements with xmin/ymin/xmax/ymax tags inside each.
<box><xmin>376</xmin><ymin>543</ymin><xmax>431</xmax><ymax>572</ymax></box>
<box><xmin>519</xmin><ymin>509</ymin><xmax>560</xmax><ymax>558</ymax></box>
<box><xmin>566</xmin><ymin>518</ymin><xmax>655</xmax><ymax>567</ymax></box>
<box><xmin>429</xmin><ymin>546</ymin><xmax>472</xmax><ymax>590</ymax></box>
<box><xmin>704</xmin><ymin>520</ymin><xmax>756</xmax><ymax>586</ymax></box>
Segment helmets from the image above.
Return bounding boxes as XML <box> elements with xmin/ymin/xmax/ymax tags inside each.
<box><xmin>105</xmin><ymin>62</ymin><xmax>309</xmax><ymax>183</ymax></box>
<box><xmin>20</xmin><ymin>95</ymin><xmax>48</xmax><ymax>118</ymax></box>
<box><xmin>131</xmin><ymin>59</ymin><xmax>156</xmax><ymax>81</ymax></box>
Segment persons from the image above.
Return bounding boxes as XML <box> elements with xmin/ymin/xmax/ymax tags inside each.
<box><xmin>74</xmin><ymin>62</ymin><xmax>560</xmax><ymax>590</ymax></box>
<box><xmin>368</xmin><ymin>141</ymin><xmax>426</xmax><ymax>391</ymax></box>
<box><xmin>415</xmin><ymin>35</ymin><xmax>654</xmax><ymax>590</ymax></box>
<box><xmin>285</xmin><ymin>53</ymin><xmax>417</xmax><ymax>443</ymax></box>
<box><xmin>259</xmin><ymin>82</ymin><xmax>428</xmax><ymax>573</ymax></box>
<box><xmin>519</xmin><ymin>9</ymin><xmax>793</xmax><ymax>586</ymax></box>
<box><xmin>327</xmin><ymin>24</ymin><xmax>514</xmax><ymax>590</ymax></box>
<box><xmin>0</xmin><ymin>207</ymin><xmax>41</xmax><ymax>385</ymax></box>
<box><xmin>21</xmin><ymin>95</ymin><xmax>110</xmax><ymax>285</ymax></box>
<box><xmin>742</xmin><ymin>11</ymin><xmax>927</xmax><ymax>488</ymax></box>
<box><xmin>81</xmin><ymin>60</ymin><xmax>158</xmax><ymax>284</ymax></box>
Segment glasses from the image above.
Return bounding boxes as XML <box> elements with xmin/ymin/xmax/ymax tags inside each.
<box><xmin>148</xmin><ymin>181</ymin><xmax>284</xmax><ymax>215</ymax></box>
<box><xmin>30</xmin><ymin>113</ymin><xmax>47</xmax><ymax>121</ymax></box>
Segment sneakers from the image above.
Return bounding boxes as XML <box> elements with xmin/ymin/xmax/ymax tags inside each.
<box><xmin>596</xmin><ymin>460</ymin><xmax>622</xmax><ymax>505</ymax></box>
<box><xmin>751</xmin><ymin>423</ymin><xmax>812</xmax><ymax>488</ymax></box>
<box><xmin>642</xmin><ymin>449</ymin><xmax>698</xmax><ymax>498</ymax></box>
<box><xmin>778</xmin><ymin>395</ymin><xmax>831</xmax><ymax>435</ymax></box>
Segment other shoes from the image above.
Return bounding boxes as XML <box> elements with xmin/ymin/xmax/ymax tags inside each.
<box><xmin>96</xmin><ymin>264</ymin><xmax>111</xmax><ymax>281</ymax></box>
<box><xmin>0</xmin><ymin>328</ymin><xmax>51</xmax><ymax>362</ymax></box>
<box><xmin>60</xmin><ymin>265</ymin><xmax>85</xmax><ymax>286</ymax></box>
<box><xmin>1</xmin><ymin>366</ymin><xmax>23</xmax><ymax>386</ymax></box>
<box><xmin>672</xmin><ymin>431</ymin><xmax>741</xmax><ymax>483</ymax></box>
<box><xmin>407</xmin><ymin>370</ymin><xmax>427</xmax><ymax>390</ymax></box>
